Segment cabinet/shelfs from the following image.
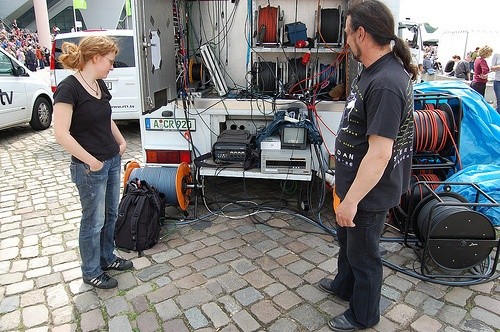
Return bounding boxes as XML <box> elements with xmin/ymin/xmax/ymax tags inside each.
<box><xmin>247</xmin><ymin>0</ymin><xmax>350</xmax><ymax>104</ymax></box>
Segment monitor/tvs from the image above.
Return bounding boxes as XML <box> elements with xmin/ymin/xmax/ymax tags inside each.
<box><xmin>280</xmin><ymin>125</ymin><xmax>307</xmax><ymax>149</ymax></box>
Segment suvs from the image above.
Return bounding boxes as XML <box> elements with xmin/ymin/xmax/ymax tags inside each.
<box><xmin>49</xmin><ymin>28</ymin><xmax>141</xmax><ymax>121</ymax></box>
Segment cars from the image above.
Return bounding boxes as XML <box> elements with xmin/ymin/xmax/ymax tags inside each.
<box><xmin>0</xmin><ymin>47</ymin><xmax>54</xmax><ymax>131</ymax></box>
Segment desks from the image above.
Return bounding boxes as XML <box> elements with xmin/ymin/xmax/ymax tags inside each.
<box><xmin>200</xmin><ymin>156</ymin><xmax>312</xmax><ymax>181</ymax></box>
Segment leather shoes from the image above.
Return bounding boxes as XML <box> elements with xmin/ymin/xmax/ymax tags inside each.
<box><xmin>326</xmin><ymin>311</ymin><xmax>363</xmax><ymax>332</ymax></box>
<box><xmin>318</xmin><ymin>275</ymin><xmax>337</xmax><ymax>295</ymax></box>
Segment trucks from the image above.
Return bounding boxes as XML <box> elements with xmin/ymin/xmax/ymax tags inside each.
<box><xmin>130</xmin><ymin>0</ymin><xmax>424</xmax><ymax>184</ymax></box>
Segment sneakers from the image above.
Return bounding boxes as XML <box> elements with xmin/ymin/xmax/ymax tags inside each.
<box><xmin>101</xmin><ymin>256</ymin><xmax>134</xmax><ymax>271</ymax></box>
<box><xmin>82</xmin><ymin>271</ymin><xmax>119</xmax><ymax>290</ymax></box>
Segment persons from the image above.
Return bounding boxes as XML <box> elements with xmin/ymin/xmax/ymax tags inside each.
<box><xmin>319</xmin><ymin>0</ymin><xmax>419</xmax><ymax>332</ymax></box>
<box><xmin>456</xmin><ymin>55</ymin><xmax>471</xmax><ymax>80</ymax></box>
<box><xmin>53</xmin><ymin>26</ymin><xmax>60</xmax><ymax>34</ymax></box>
<box><xmin>53</xmin><ymin>35</ymin><xmax>133</xmax><ymax>289</ymax></box>
<box><xmin>491</xmin><ymin>52</ymin><xmax>500</xmax><ymax>114</ymax></box>
<box><xmin>71</xmin><ymin>26</ymin><xmax>75</xmax><ymax>32</ymax></box>
<box><xmin>0</xmin><ymin>19</ymin><xmax>50</xmax><ymax>72</ymax></box>
<box><xmin>469</xmin><ymin>47</ymin><xmax>480</xmax><ymax>71</ymax></box>
<box><xmin>470</xmin><ymin>45</ymin><xmax>493</xmax><ymax>97</ymax></box>
<box><xmin>444</xmin><ymin>55</ymin><xmax>460</xmax><ymax>76</ymax></box>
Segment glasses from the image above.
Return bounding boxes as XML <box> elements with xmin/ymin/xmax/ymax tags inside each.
<box><xmin>107</xmin><ymin>57</ymin><xmax>116</xmax><ymax>65</ymax></box>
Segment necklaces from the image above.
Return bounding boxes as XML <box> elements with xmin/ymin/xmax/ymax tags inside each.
<box><xmin>78</xmin><ymin>70</ymin><xmax>98</xmax><ymax>95</ymax></box>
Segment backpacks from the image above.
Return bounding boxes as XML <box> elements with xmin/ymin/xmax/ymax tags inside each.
<box><xmin>111</xmin><ymin>176</ymin><xmax>167</xmax><ymax>259</ymax></box>
